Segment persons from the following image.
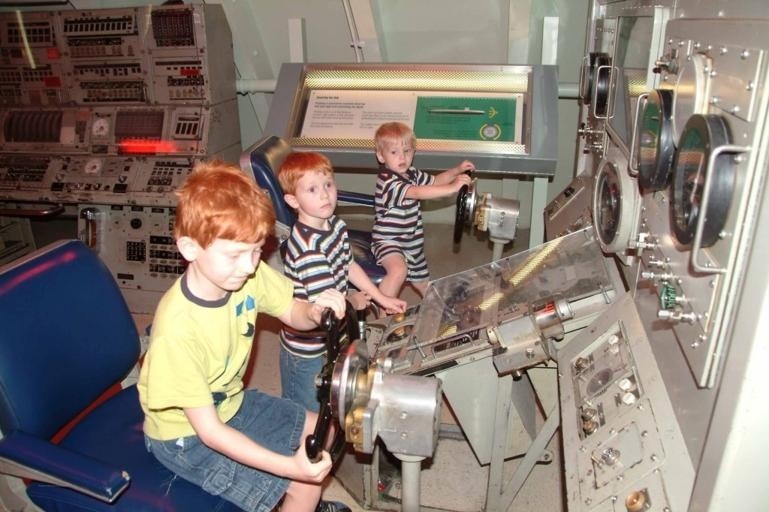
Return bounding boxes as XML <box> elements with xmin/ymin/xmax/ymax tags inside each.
<box><xmin>278</xmin><ymin>151</ymin><xmax>407</xmax><ymax>414</ymax></box>
<box><xmin>370</xmin><ymin>122</ymin><xmax>475</xmax><ymax>318</ymax></box>
<box><xmin>136</xmin><ymin>159</ymin><xmax>351</xmax><ymax>512</ymax></box>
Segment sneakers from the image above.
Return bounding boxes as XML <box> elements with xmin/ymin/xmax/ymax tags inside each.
<box><xmin>315</xmin><ymin>501</ymin><xmax>350</xmax><ymax>512</ymax></box>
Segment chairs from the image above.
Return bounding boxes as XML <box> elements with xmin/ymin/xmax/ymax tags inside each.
<box><xmin>238</xmin><ymin>135</ymin><xmax>389</xmax><ymax>340</ymax></box>
<box><xmin>1</xmin><ymin>239</ymin><xmax>287</xmax><ymax>512</ymax></box>
